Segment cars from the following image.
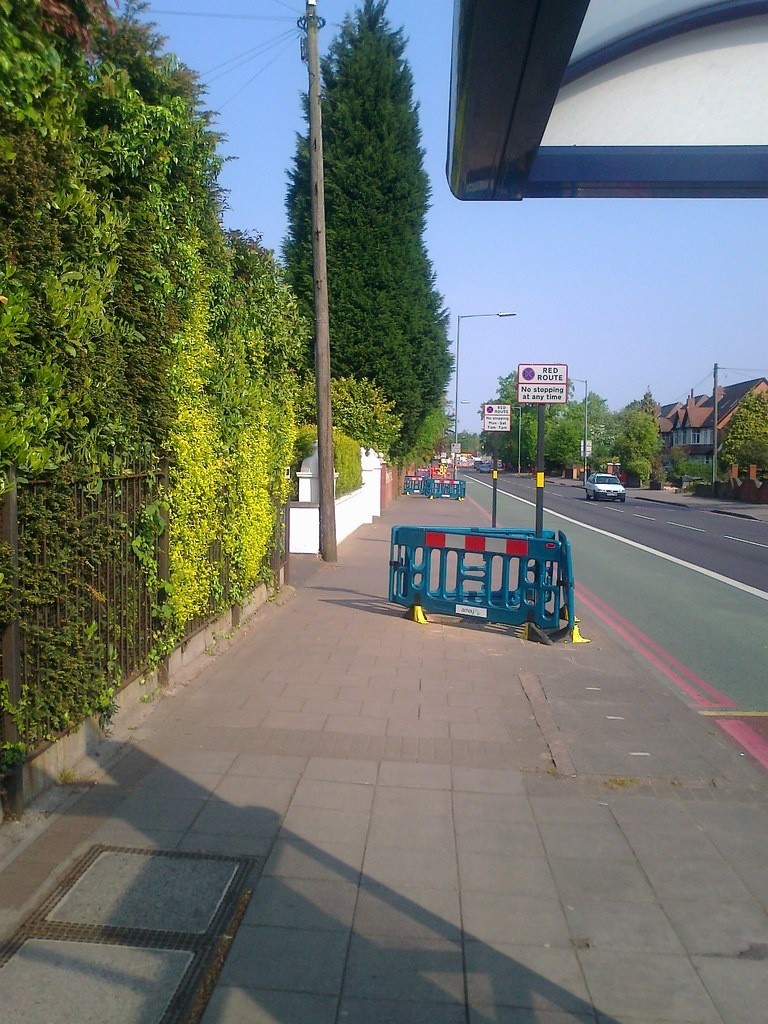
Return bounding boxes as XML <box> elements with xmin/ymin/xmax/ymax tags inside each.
<box><xmin>459</xmin><ymin>455</ymin><xmax>505</xmax><ymax>474</ymax></box>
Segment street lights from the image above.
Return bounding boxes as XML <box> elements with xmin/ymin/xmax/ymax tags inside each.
<box><xmin>460</xmin><ymin>400</ymin><xmax>491</xmax><ymax>404</ymax></box>
<box><xmin>452</xmin><ymin>312</ymin><xmax>517</xmax><ymax>480</ymax></box>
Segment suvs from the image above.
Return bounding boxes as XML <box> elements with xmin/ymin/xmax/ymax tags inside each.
<box><xmin>585</xmin><ymin>472</ymin><xmax>626</xmax><ymax>502</ymax></box>
